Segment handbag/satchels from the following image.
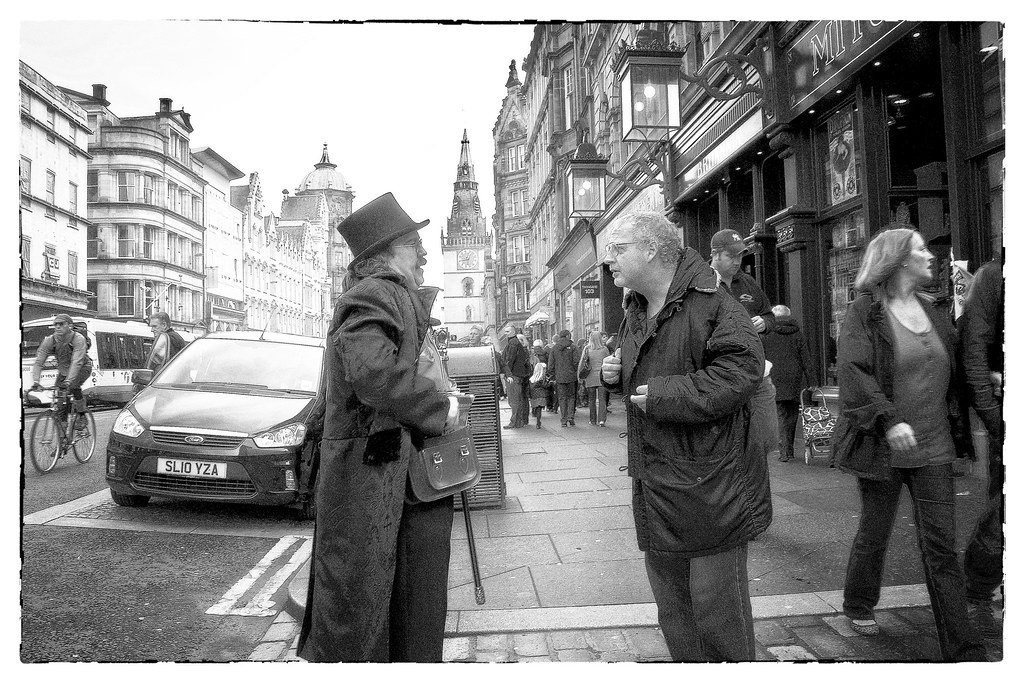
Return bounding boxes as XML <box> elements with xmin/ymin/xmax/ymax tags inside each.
<box><xmin>579</xmin><ymin>349</ymin><xmax>592</xmax><ymax>379</ymax></box>
<box><xmin>404</xmin><ymin>395</ymin><xmax>482</xmax><ymax>504</ymax></box>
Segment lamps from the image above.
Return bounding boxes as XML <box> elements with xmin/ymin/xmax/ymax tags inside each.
<box><xmin>562</xmin><ymin>129</ymin><xmax>672</xmax><ymax>219</ymax></box>
<box><xmin>610</xmin><ymin>21</ymin><xmax>771</xmax><ymax>142</ymax></box>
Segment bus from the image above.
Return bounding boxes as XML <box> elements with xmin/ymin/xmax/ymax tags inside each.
<box><xmin>22</xmin><ymin>314</ymin><xmax>202</xmax><ymax>409</ymax></box>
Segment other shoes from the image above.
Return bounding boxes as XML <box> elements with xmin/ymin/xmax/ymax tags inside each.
<box><xmin>778</xmin><ymin>454</ymin><xmax>788</xmax><ymax>461</ymax></box>
<box><xmin>787</xmin><ymin>449</ymin><xmax>794</xmax><ymax>458</ymax></box>
<box><xmin>561</xmin><ymin>421</ymin><xmax>568</xmax><ymax>428</ymax></box>
<box><xmin>569</xmin><ymin>420</ymin><xmax>575</xmax><ymax>426</ymax></box>
<box><xmin>967</xmin><ymin>597</ymin><xmax>1000</xmax><ymax>638</ymax></box>
<box><xmin>599</xmin><ymin>421</ymin><xmax>605</xmax><ymax>427</ymax></box>
<box><xmin>75</xmin><ymin>416</ymin><xmax>89</xmax><ymax>430</ymax></box>
<box><xmin>51</xmin><ymin>448</ymin><xmax>67</xmax><ymax>457</ymax></box>
<box><xmin>503</xmin><ymin>421</ymin><xmax>528</xmax><ymax>428</ymax></box>
<box><xmin>536</xmin><ymin>422</ymin><xmax>541</xmax><ymax>429</ymax></box>
<box><xmin>589</xmin><ymin>421</ymin><xmax>596</xmax><ymax>425</ymax></box>
<box><xmin>848</xmin><ymin>616</ymin><xmax>880</xmax><ymax>637</ymax></box>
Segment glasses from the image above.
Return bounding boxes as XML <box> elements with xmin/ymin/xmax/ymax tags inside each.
<box><xmin>385</xmin><ymin>238</ymin><xmax>425</xmax><ymax>255</ymax></box>
<box><xmin>604</xmin><ymin>240</ymin><xmax>644</xmax><ymax>258</ymax></box>
<box><xmin>53</xmin><ymin>321</ymin><xmax>66</xmax><ymax>327</ymax></box>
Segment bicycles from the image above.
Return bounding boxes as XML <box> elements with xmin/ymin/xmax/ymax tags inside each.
<box><xmin>27</xmin><ymin>385</ymin><xmax>97</xmax><ymax>474</ymax></box>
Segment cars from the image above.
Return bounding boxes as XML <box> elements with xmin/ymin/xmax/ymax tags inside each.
<box><xmin>104</xmin><ymin>331</ymin><xmax>327</xmax><ymax>520</ymax></box>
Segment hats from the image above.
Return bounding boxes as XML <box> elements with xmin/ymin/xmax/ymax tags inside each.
<box><xmin>55</xmin><ymin>313</ymin><xmax>78</xmax><ymax>329</ymax></box>
<box><xmin>337</xmin><ymin>192</ymin><xmax>430</xmax><ymax>272</ymax></box>
<box><xmin>710</xmin><ymin>229</ymin><xmax>748</xmax><ymax>255</ymax></box>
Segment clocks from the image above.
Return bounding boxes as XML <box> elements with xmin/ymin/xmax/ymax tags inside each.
<box><xmin>457</xmin><ymin>248</ymin><xmax>478</xmax><ymax>269</ymax></box>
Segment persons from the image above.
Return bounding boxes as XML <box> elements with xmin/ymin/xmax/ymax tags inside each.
<box><xmin>956</xmin><ymin>257</ymin><xmax>1004</xmax><ymax>639</ymax></box>
<box><xmin>707</xmin><ymin>230</ymin><xmax>780</xmax><ymax>468</ymax></box>
<box><xmin>763</xmin><ymin>305</ymin><xmax>817</xmax><ymax>462</ymax></box>
<box><xmin>32</xmin><ymin>314</ymin><xmax>93</xmax><ymax>457</ymax></box>
<box><xmin>295</xmin><ymin>192</ymin><xmax>475</xmax><ymax>663</ymax></box>
<box><xmin>143</xmin><ymin>311</ymin><xmax>185</xmax><ymax>388</ymax></box>
<box><xmin>463</xmin><ymin>325</ymin><xmax>484</xmax><ymax>347</ymax></box>
<box><xmin>577</xmin><ymin>331</ymin><xmax>614</xmax><ymax>427</ymax></box>
<box><xmin>829</xmin><ymin>228</ymin><xmax>989</xmax><ymax>661</ymax></box>
<box><xmin>599</xmin><ymin>210</ymin><xmax>771</xmax><ymax>661</ymax></box>
<box><xmin>531</xmin><ymin>330</ymin><xmax>582</xmax><ymax>429</ymax></box>
<box><xmin>502</xmin><ymin>325</ymin><xmax>533</xmax><ymax>429</ymax></box>
<box><xmin>481</xmin><ymin>335</ymin><xmax>514</xmax><ymax>400</ymax></box>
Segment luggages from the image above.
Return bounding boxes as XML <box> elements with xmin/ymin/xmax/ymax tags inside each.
<box><xmin>800</xmin><ymin>386</ymin><xmax>837</xmax><ymax>465</ymax></box>
